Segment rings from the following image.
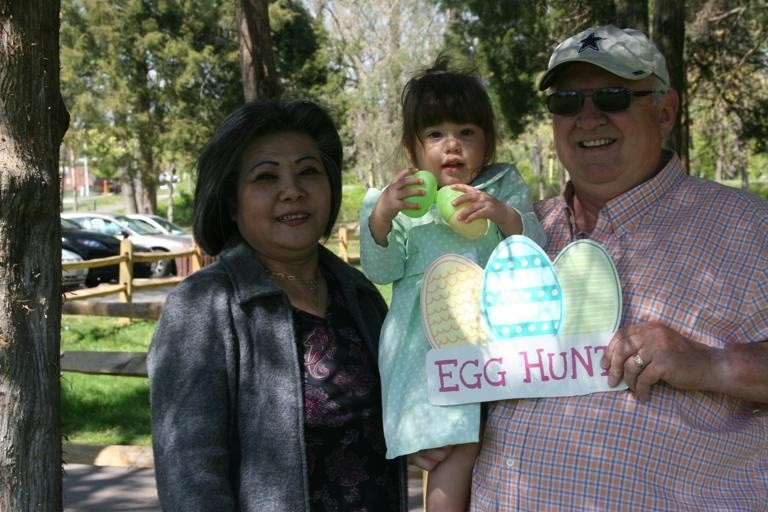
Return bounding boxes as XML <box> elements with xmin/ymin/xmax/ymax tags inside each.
<box><xmin>632</xmin><ymin>354</ymin><xmax>646</xmax><ymax>367</ymax></box>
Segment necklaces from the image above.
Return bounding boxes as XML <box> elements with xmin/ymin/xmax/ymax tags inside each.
<box><xmin>265</xmin><ymin>264</ymin><xmax>327</xmax><ymax>306</ymax></box>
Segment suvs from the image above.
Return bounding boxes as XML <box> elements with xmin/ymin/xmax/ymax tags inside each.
<box><xmin>63</xmin><ymin>213</ymin><xmax>195</xmax><ymax>279</ymax></box>
<box><xmin>58</xmin><ymin>216</ymin><xmax>126</xmax><ymax>289</ymax></box>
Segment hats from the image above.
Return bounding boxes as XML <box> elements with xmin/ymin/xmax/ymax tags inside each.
<box><xmin>538</xmin><ymin>25</ymin><xmax>670</xmax><ymax>91</ymax></box>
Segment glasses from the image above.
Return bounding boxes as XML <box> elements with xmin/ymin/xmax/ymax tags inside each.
<box><xmin>546</xmin><ymin>87</ymin><xmax>654</xmax><ymax>116</ymax></box>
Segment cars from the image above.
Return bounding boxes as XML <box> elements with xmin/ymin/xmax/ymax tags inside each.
<box><xmin>57</xmin><ymin>247</ymin><xmax>89</xmax><ymax>290</ymax></box>
<box><xmin>158</xmin><ymin>172</ymin><xmax>176</xmax><ymax>185</ymax></box>
<box><xmin>129</xmin><ymin>214</ymin><xmax>194</xmax><ymax>240</ymax></box>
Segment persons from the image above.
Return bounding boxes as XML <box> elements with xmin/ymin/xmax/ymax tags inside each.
<box><xmin>146</xmin><ymin>97</ymin><xmax>409</xmax><ymax>510</ymax></box>
<box><xmin>357</xmin><ymin>68</ymin><xmax>550</xmax><ymax>511</ymax></box>
<box><xmin>409</xmin><ymin>21</ymin><xmax>767</xmax><ymax>511</ymax></box>
